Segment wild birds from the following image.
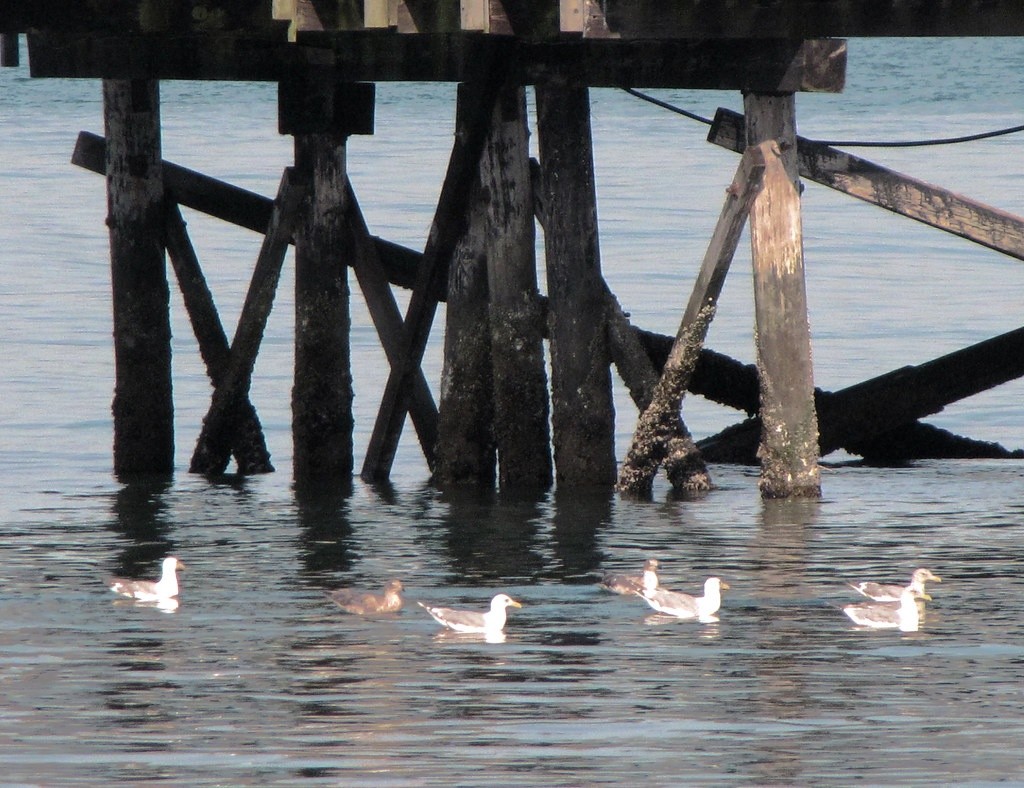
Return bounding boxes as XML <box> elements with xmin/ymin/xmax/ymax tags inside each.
<box><xmin>586</xmin><ymin>559</ymin><xmax>732</xmax><ymax>618</ymax></box>
<box><xmin>825</xmin><ymin>568</ymin><xmax>943</xmax><ymax>633</ymax></box>
<box><xmin>318</xmin><ymin>579</ymin><xmax>407</xmax><ymax>616</ymax></box>
<box><xmin>416</xmin><ymin>594</ymin><xmax>523</xmax><ymax>635</ymax></box>
<box><xmin>93</xmin><ymin>556</ymin><xmax>187</xmax><ymax>603</ymax></box>
